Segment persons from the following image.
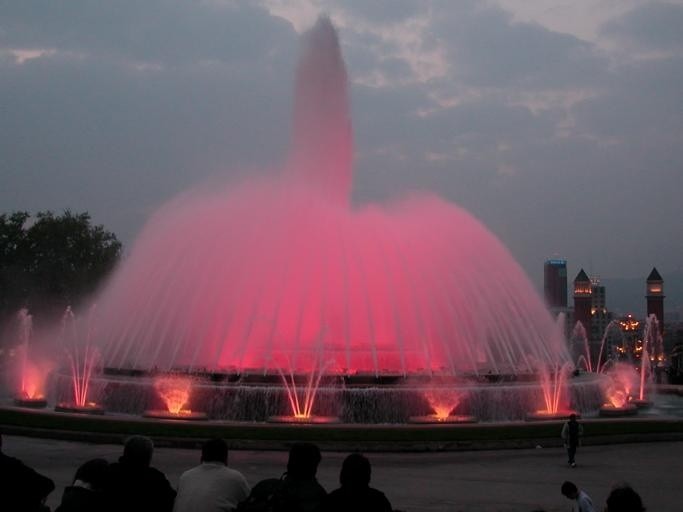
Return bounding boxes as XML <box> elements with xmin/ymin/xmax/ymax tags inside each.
<box><xmin>99</xmin><ymin>435</ymin><xmax>177</xmax><ymax>511</ymax></box>
<box><xmin>55</xmin><ymin>457</ymin><xmax>111</xmax><ymax>511</ymax></box>
<box><xmin>560</xmin><ymin>479</ymin><xmax>592</xmax><ymax>512</ymax></box>
<box><xmin>561</xmin><ymin>413</ymin><xmax>583</xmax><ymax>468</ymax></box>
<box><xmin>323</xmin><ymin>452</ymin><xmax>392</xmax><ymax>512</ymax></box>
<box><xmin>0</xmin><ymin>434</ymin><xmax>56</xmax><ymax>511</ymax></box>
<box><xmin>604</xmin><ymin>478</ymin><xmax>645</xmax><ymax>512</ymax></box>
<box><xmin>172</xmin><ymin>436</ymin><xmax>253</xmax><ymax>511</ymax></box>
<box><xmin>232</xmin><ymin>442</ymin><xmax>328</xmax><ymax>511</ymax></box>
